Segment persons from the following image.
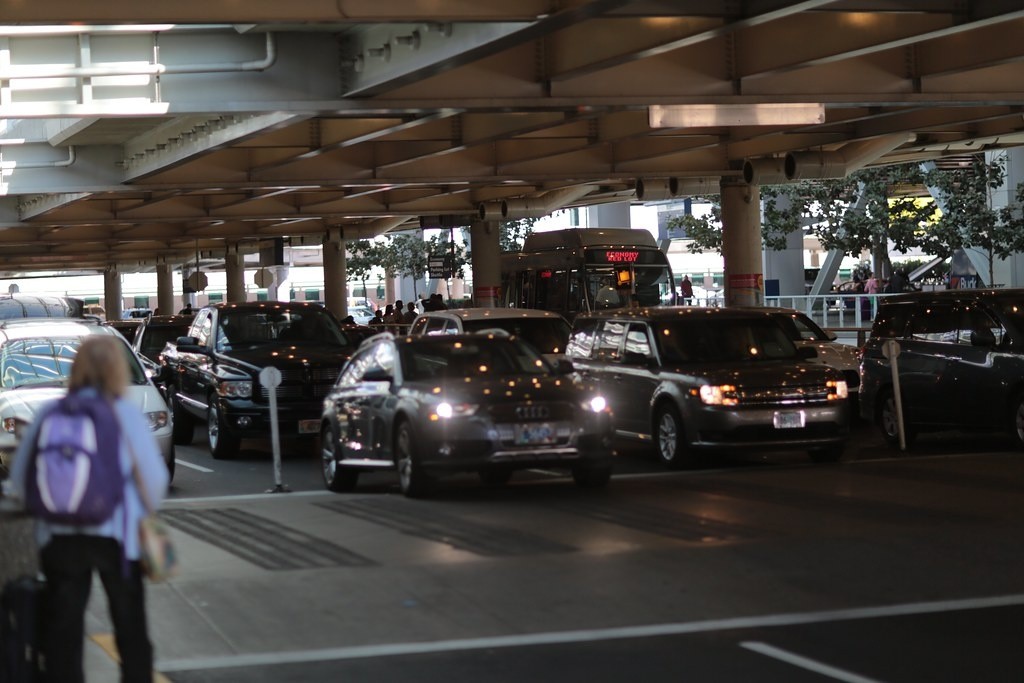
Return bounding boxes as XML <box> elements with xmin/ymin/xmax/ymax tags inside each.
<box><xmin>891</xmin><ymin>268</ymin><xmax>906</xmax><ymax>293</ymax></box>
<box><xmin>854</xmin><ymin>269</ymin><xmax>869</xmax><ymax>294</ymax></box>
<box><xmin>864</xmin><ymin>272</ymin><xmax>880</xmax><ymax>322</ymax></box>
<box><xmin>422</xmin><ymin>293</ymin><xmax>447</xmax><ymax>312</ymax></box>
<box><xmin>341</xmin><ymin>315</ymin><xmax>356</xmax><ymax>325</ymax></box>
<box><xmin>183</xmin><ymin>303</ymin><xmax>195</xmax><ymax>315</ymax></box>
<box><xmin>393</xmin><ymin>300</ymin><xmax>404</xmax><ymax>323</ymax></box>
<box><xmin>680</xmin><ymin>275</ymin><xmax>693</xmax><ymax>298</ymax></box>
<box><xmin>371</xmin><ymin>310</ymin><xmax>382</xmax><ymax>323</ymax></box>
<box><xmin>11</xmin><ymin>335</ymin><xmax>171</xmax><ymax>683</ymax></box>
<box><xmin>404</xmin><ymin>302</ymin><xmax>418</xmax><ymax>324</ymax></box>
<box><xmin>595</xmin><ymin>278</ymin><xmax>629</xmax><ymax>309</ymax></box>
<box><xmin>383</xmin><ymin>304</ymin><xmax>395</xmax><ymax>323</ymax></box>
<box><xmin>416</xmin><ymin>294</ymin><xmax>424</xmax><ymax>314</ymax></box>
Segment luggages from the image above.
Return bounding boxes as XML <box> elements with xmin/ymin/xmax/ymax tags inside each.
<box><xmin>0</xmin><ymin>570</ymin><xmax>52</xmax><ymax>683</ymax></box>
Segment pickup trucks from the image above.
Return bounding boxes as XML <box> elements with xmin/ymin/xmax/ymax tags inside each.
<box><xmin>162</xmin><ymin>300</ymin><xmax>356</xmax><ymax>460</ymax></box>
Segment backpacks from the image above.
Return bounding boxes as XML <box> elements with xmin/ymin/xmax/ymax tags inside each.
<box><xmin>25</xmin><ymin>395</ymin><xmax>131</xmax><ymax>526</ymax></box>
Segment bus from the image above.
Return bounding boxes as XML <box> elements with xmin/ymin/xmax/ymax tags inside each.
<box><xmin>510</xmin><ymin>227</ymin><xmax>679</xmax><ymax>314</ymax></box>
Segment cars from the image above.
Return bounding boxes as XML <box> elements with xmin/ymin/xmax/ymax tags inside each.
<box><xmin>0</xmin><ymin>318</ymin><xmax>176</xmax><ymax>490</ymax></box>
<box><xmin>827</xmin><ymin>281</ymin><xmax>865</xmax><ymax>303</ymax></box>
<box><xmin>409</xmin><ymin>307</ymin><xmax>576</xmax><ymax>369</ymax></box>
<box><xmin>1</xmin><ymin>292</ymin><xmax>154</xmax><ymax>327</ymax></box>
<box><xmin>728</xmin><ymin>305</ymin><xmax>860</xmax><ymax>394</ymax></box>
<box><xmin>319</xmin><ymin>326</ymin><xmax>621</xmax><ymax>498</ymax></box>
<box><xmin>131</xmin><ymin>312</ymin><xmax>196</xmax><ymax>383</ymax></box>
<box><xmin>346</xmin><ymin>297</ymin><xmax>385</xmax><ymax>328</ymax></box>
<box><xmin>674</xmin><ymin>281</ymin><xmax>729</xmax><ymax>310</ymax></box>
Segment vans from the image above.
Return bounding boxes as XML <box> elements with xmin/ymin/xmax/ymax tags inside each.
<box><xmin>856</xmin><ymin>289</ymin><xmax>1023</xmax><ymax>452</ymax></box>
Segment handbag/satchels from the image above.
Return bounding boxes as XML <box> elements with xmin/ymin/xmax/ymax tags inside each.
<box><xmin>139</xmin><ymin>517</ymin><xmax>174</xmax><ymax>581</ymax></box>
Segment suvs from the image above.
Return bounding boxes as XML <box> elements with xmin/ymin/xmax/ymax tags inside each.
<box><xmin>569</xmin><ymin>304</ymin><xmax>851</xmax><ymax>473</ymax></box>
